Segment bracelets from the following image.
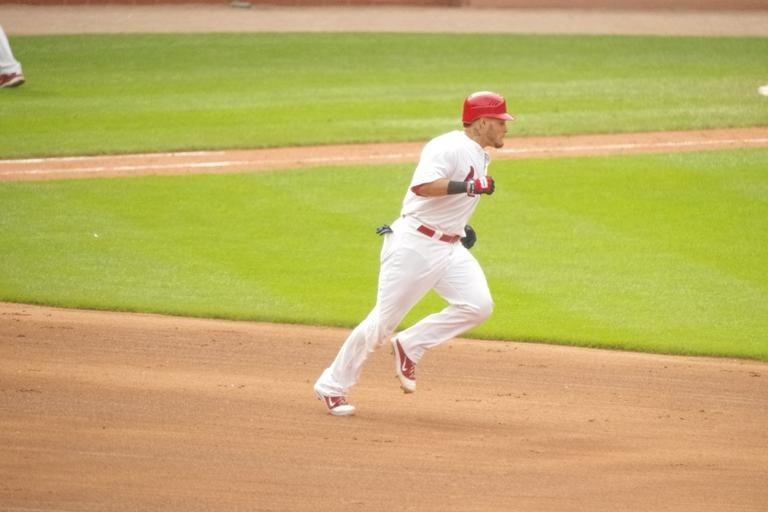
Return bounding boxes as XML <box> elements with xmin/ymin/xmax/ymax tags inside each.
<box><xmin>447</xmin><ymin>180</ymin><xmax>468</xmax><ymax>193</ymax></box>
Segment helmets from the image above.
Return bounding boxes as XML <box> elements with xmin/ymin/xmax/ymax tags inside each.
<box><xmin>463</xmin><ymin>90</ymin><xmax>514</xmax><ymax>125</ymax></box>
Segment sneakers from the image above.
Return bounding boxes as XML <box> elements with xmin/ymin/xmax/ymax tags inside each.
<box><xmin>1</xmin><ymin>70</ymin><xmax>25</xmax><ymax>88</ymax></box>
<box><xmin>313</xmin><ymin>387</ymin><xmax>356</xmax><ymax>416</ymax></box>
<box><xmin>390</xmin><ymin>336</ymin><xmax>416</xmax><ymax>394</ymax></box>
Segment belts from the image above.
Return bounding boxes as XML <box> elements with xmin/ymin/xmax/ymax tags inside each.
<box><xmin>417</xmin><ymin>225</ymin><xmax>460</xmax><ymax>245</ymax></box>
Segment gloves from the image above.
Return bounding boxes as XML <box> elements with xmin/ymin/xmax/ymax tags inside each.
<box><xmin>466</xmin><ymin>175</ymin><xmax>494</xmax><ymax>196</ymax></box>
<box><xmin>376</xmin><ymin>224</ymin><xmax>393</xmax><ymax>237</ymax></box>
<box><xmin>461</xmin><ymin>225</ymin><xmax>476</xmax><ymax>249</ymax></box>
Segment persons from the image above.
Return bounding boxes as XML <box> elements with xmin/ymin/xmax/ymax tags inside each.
<box><xmin>0</xmin><ymin>23</ymin><xmax>27</xmax><ymax>88</ymax></box>
<box><xmin>312</xmin><ymin>90</ymin><xmax>515</xmax><ymax>416</ymax></box>
